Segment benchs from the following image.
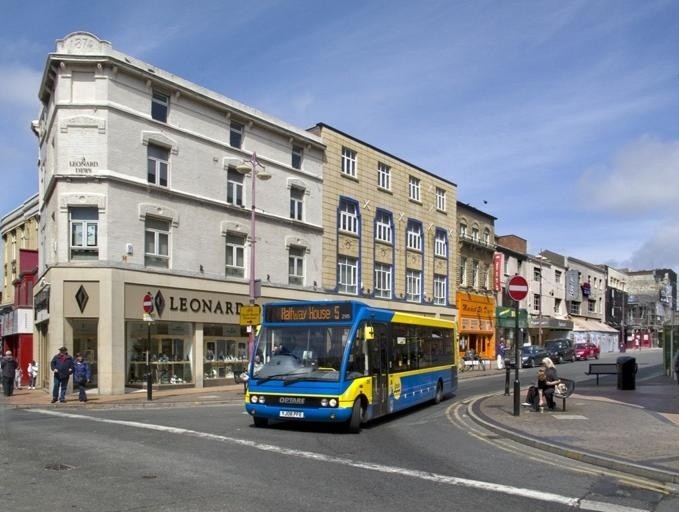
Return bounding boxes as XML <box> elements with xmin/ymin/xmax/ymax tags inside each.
<box><xmin>554</xmin><ymin>378</ymin><xmax>575</xmax><ymax>412</ymax></box>
<box><xmin>585</xmin><ymin>363</ymin><xmax>617</xmax><ymax>385</ymax></box>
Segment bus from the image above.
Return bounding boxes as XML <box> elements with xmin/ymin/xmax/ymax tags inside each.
<box><xmin>242</xmin><ymin>296</ymin><xmax>460</xmax><ymax>435</ymax></box>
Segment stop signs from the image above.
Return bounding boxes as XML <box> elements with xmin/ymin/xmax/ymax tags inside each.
<box><xmin>142</xmin><ymin>294</ymin><xmax>154</xmax><ymax>313</ymax></box>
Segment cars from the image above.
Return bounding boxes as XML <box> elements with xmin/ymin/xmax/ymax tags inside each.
<box><xmin>504</xmin><ymin>336</ymin><xmax>601</xmax><ymax>370</ymax></box>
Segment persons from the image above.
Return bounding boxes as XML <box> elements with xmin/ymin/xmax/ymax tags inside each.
<box><xmin>26</xmin><ymin>360</ymin><xmax>39</xmax><ymax>390</ymax></box>
<box><xmin>69</xmin><ymin>352</ymin><xmax>92</xmax><ymax>402</ymax></box>
<box><xmin>248</xmin><ymin>355</ymin><xmax>264</xmax><ymax>378</ymax></box>
<box><xmin>274</xmin><ymin>333</ymin><xmax>301</xmax><ymax>362</ymax></box>
<box><xmin>519</xmin><ymin>356</ymin><xmax>561</xmax><ymax>406</ymax></box>
<box><xmin>536</xmin><ymin>367</ymin><xmax>560</xmax><ymax>409</ymax></box>
<box><xmin>49</xmin><ymin>347</ymin><xmax>75</xmax><ymax>403</ymax></box>
<box><xmin>14</xmin><ymin>366</ymin><xmax>25</xmax><ymax>390</ymax></box>
<box><xmin>496</xmin><ymin>338</ymin><xmax>511</xmax><ymax>369</ymax></box>
<box><xmin>0</xmin><ymin>350</ymin><xmax>19</xmax><ymax>396</ymax></box>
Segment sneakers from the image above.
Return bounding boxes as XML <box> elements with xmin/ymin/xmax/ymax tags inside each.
<box><xmin>51</xmin><ymin>400</ymin><xmax>63</xmax><ymax>403</ymax></box>
<box><xmin>521</xmin><ymin>402</ymin><xmax>529</xmax><ymax>405</ymax></box>
<box><xmin>28</xmin><ymin>387</ymin><xmax>35</xmax><ymax>390</ymax></box>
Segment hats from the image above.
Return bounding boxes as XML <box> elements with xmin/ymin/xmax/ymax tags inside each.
<box><xmin>59</xmin><ymin>347</ymin><xmax>66</xmax><ymax>350</ymax></box>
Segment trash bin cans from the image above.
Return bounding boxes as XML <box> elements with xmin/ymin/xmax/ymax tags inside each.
<box><xmin>616</xmin><ymin>356</ymin><xmax>635</xmax><ymax>390</ymax></box>
